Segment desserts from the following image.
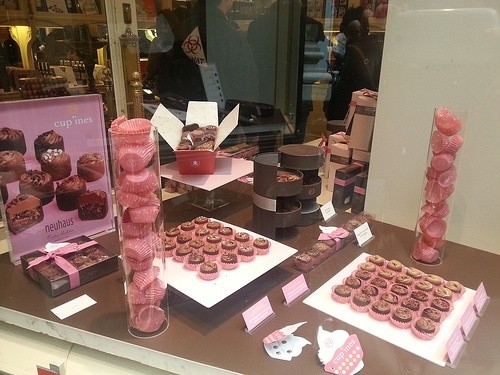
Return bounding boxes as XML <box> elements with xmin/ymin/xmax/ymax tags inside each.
<box><xmin>0</xmin><ymin>126</ymin><xmax>109</xmax><ymax>234</ymax></box>
<box><xmin>27</xmin><ymin>247</ymin><xmax>102</xmax><ymax>279</ymax></box>
<box><xmin>297</xmin><ymin>215</ymin><xmax>372</xmax><ymax>264</ymax></box>
<box><xmin>157</xmin><ymin>216</ymin><xmax>271</xmax><ymax>280</ymax></box>
<box><xmin>332</xmin><ymin>254</ymin><xmax>465</xmax><ymax>339</ymax></box>
<box><xmin>177</xmin><ymin>123</ymin><xmax>216</xmax><ymax>150</ymax></box>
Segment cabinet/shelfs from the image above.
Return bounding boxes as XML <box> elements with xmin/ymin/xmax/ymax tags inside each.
<box><xmin>253</xmin><ymin>143</ymin><xmax>324</xmax><ymax>240</ymax></box>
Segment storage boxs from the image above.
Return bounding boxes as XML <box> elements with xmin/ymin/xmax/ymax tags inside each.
<box><xmin>345</xmin><ymin>95</ymin><xmax>378</xmax><ymax>152</ymax></box>
<box><xmin>324</xmin><ymin>132</ymin><xmax>349</xmax><ymax>179</ymax></box>
<box><xmin>332</xmin><ymin>161</ymin><xmax>368</xmax><ymax>210</ymax></box>
<box><xmin>341</xmin><ymin>87</ymin><xmax>378</xmax><ymax>135</ymax></box>
<box><xmin>327</xmin><ymin>142</ymin><xmax>352</xmax><ymax>192</ymax></box>
<box><xmin>292</xmin><ymin>209</ymin><xmax>376</xmax><ymax>274</ymax></box>
<box><xmin>20</xmin><ymin>234</ymin><xmax>120</xmax><ymax>298</ymax></box>
<box><xmin>151</xmin><ymin>100</ymin><xmax>240</xmax><ymax>175</ymax></box>
<box><xmin>351</xmin><ymin>169</ymin><xmax>370</xmax><ymax>214</ymax></box>
<box><xmin>351</xmin><ymin>150</ymin><xmax>371</xmax><ymax>163</ymax></box>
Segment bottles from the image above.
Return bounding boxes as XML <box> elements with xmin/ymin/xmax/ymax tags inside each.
<box><xmin>20</xmin><ymin>59</ymin><xmax>87</xmax><ymax>99</ymax></box>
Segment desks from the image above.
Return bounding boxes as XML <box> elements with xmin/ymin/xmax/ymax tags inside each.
<box><xmin>0</xmin><ymin>179</ymin><xmax>500</xmax><ymax>375</ymax></box>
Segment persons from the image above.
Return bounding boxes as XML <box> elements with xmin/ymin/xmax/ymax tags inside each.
<box><xmin>0</xmin><ymin>27</ymin><xmax>23</xmax><ymax>91</ymax></box>
<box><xmin>32</xmin><ymin>0</ymin><xmax>376</xmax><ymax>148</ymax></box>
